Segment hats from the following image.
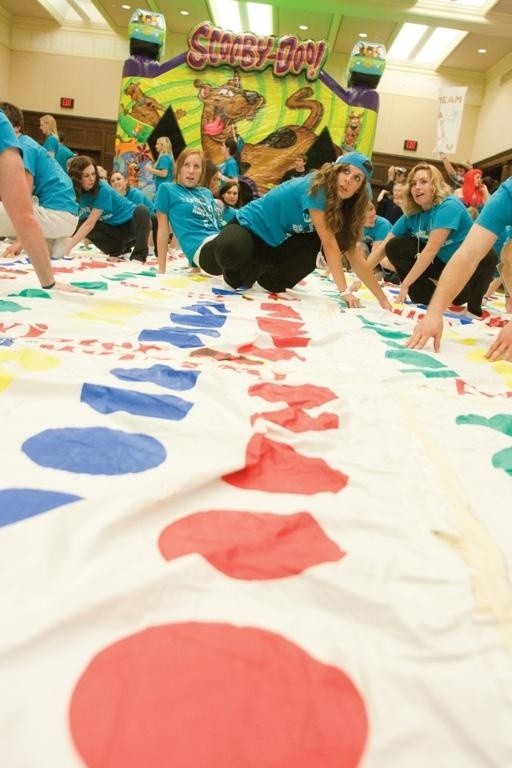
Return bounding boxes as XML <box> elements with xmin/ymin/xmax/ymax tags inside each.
<box><xmin>335</xmin><ymin>151</ymin><xmax>374</xmax><ymax>183</ymax></box>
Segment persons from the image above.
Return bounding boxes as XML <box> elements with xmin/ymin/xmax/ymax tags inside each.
<box><xmin>215</xmin><ymin>152</ymin><xmax>393</xmax><ymax>313</ymax></box>
<box><xmin>358</xmin><ymin>151</ymin><xmax>512</xmax><ymax>364</ymax></box>
<box><xmin>0</xmin><ymin>101</ymin><xmax>241</xmax><ymax>294</ymax></box>
